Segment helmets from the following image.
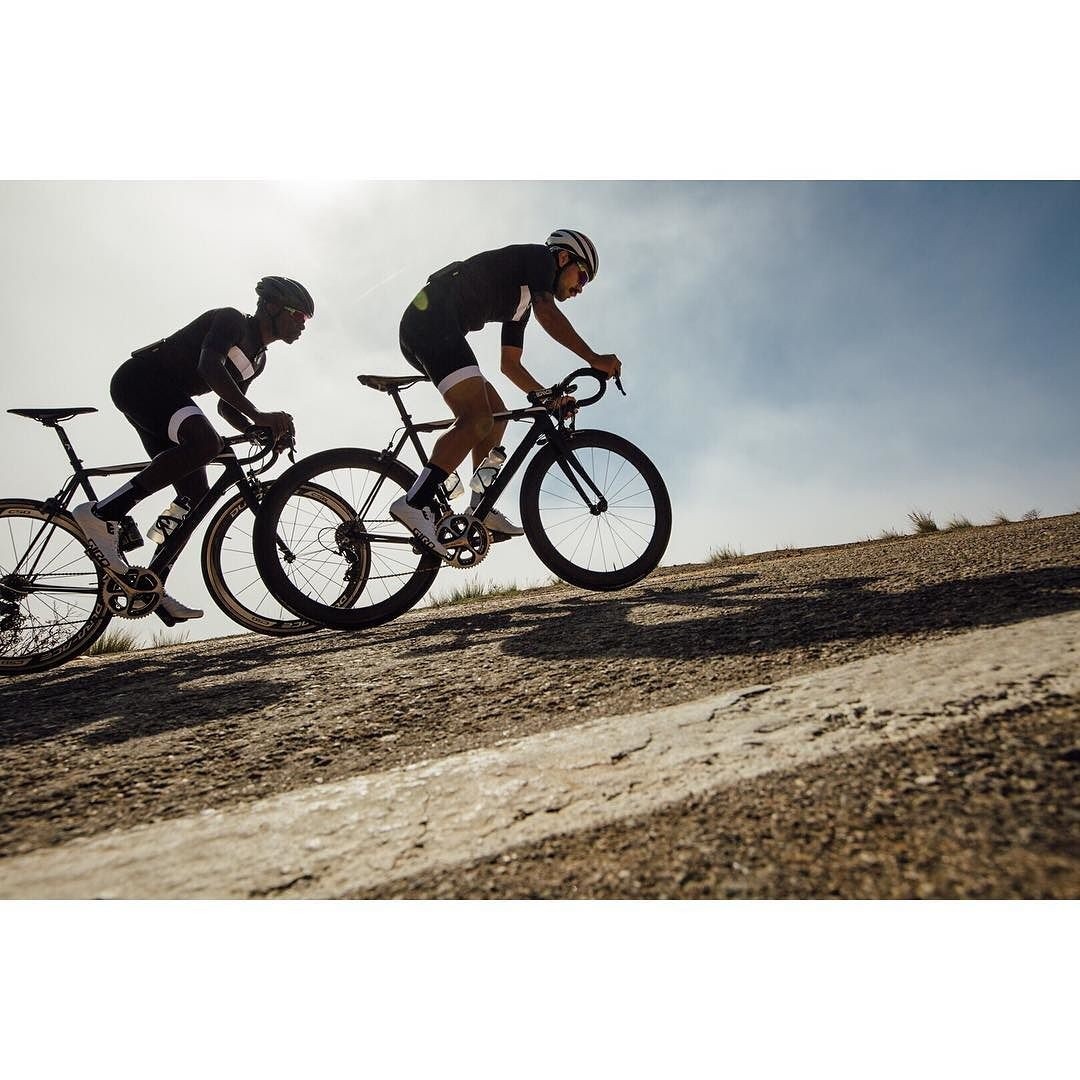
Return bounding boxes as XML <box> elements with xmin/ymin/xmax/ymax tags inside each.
<box><xmin>546</xmin><ymin>230</ymin><xmax>599</xmax><ymax>284</ymax></box>
<box><xmin>256</xmin><ymin>276</ymin><xmax>315</xmax><ymax>320</ymax></box>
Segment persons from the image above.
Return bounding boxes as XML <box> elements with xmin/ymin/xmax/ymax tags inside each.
<box><xmin>71</xmin><ymin>276</ymin><xmax>314</xmax><ymax>619</ymax></box>
<box><xmin>390</xmin><ymin>229</ymin><xmax>622</xmax><ymax>556</ymax></box>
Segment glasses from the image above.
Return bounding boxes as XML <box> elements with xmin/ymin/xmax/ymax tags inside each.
<box><xmin>570</xmin><ymin>252</ymin><xmax>590</xmax><ymax>288</ymax></box>
<box><xmin>278</xmin><ymin>304</ymin><xmax>306</xmax><ymax>324</ymax></box>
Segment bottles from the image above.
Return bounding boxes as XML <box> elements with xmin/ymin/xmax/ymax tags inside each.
<box><xmin>116</xmin><ymin>516</ymin><xmax>144</xmax><ymax>552</ymax></box>
<box><xmin>147</xmin><ymin>496</ymin><xmax>191</xmax><ymax>544</ymax></box>
<box><xmin>469</xmin><ymin>445</ymin><xmax>507</xmax><ymax>494</ymax></box>
<box><xmin>444</xmin><ymin>469</ymin><xmax>465</xmax><ymax>501</ymax></box>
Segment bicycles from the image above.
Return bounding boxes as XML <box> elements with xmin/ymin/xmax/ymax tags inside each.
<box><xmin>0</xmin><ymin>407</ymin><xmax>372</xmax><ymax>677</ymax></box>
<box><xmin>252</xmin><ymin>367</ymin><xmax>673</xmax><ymax>633</ymax></box>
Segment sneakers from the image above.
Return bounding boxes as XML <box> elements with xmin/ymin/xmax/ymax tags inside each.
<box><xmin>132</xmin><ymin>591</ymin><xmax>204</xmax><ymax>619</ymax></box>
<box><xmin>72</xmin><ymin>500</ymin><xmax>129</xmax><ymax>575</ymax></box>
<box><xmin>462</xmin><ymin>506</ymin><xmax>525</xmax><ymax>536</ymax></box>
<box><xmin>389</xmin><ymin>496</ymin><xmax>447</xmax><ymax>557</ymax></box>
